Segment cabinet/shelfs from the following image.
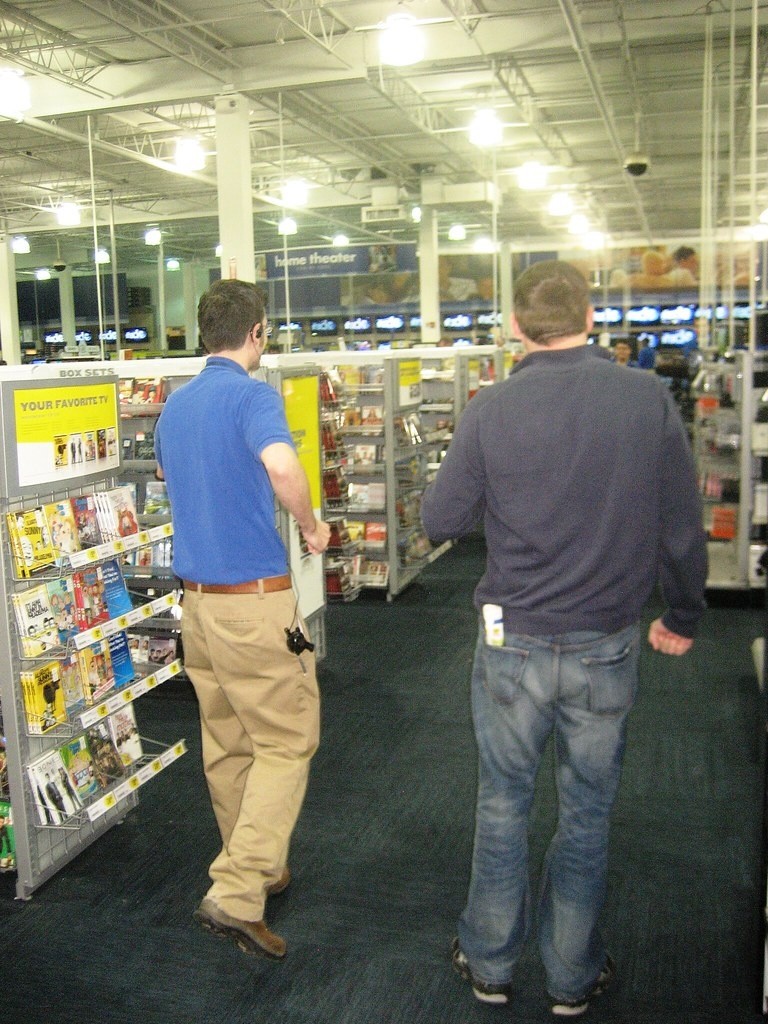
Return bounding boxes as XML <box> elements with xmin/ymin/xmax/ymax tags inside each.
<box><xmin>689</xmin><ymin>344</ymin><xmax>768</xmax><ymax>590</ymax></box>
<box><xmin>0</xmin><ymin>345</ymin><xmax>502</xmax><ymax>905</ymax></box>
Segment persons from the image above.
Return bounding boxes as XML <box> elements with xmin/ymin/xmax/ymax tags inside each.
<box><xmin>610</xmin><ymin>338</ymin><xmax>655</xmax><ymax>372</ymax></box>
<box><xmin>641</xmin><ymin>247</ymin><xmax>698</xmax><ymax>287</ymax></box>
<box><xmin>420</xmin><ymin>260</ymin><xmax>707</xmax><ymax>1014</ymax></box>
<box><xmin>155</xmin><ymin>279</ymin><xmax>330</xmax><ymax>959</ymax></box>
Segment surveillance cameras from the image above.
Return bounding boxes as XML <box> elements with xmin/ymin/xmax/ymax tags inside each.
<box><xmin>625</xmin><ymin>152</ymin><xmax>650</xmax><ymax>175</ymax></box>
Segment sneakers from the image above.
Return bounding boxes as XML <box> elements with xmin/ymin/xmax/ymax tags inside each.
<box><xmin>549</xmin><ymin>952</ymin><xmax>618</xmax><ymax>1017</ymax></box>
<box><xmin>450</xmin><ymin>936</ymin><xmax>512</xmax><ymax>1004</ymax></box>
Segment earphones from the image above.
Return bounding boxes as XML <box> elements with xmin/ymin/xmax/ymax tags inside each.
<box><xmin>256</xmin><ymin>327</ymin><xmax>263</xmax><ymax>338</ymax></box>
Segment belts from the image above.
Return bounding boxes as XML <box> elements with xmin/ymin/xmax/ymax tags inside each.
<box><xmin>182</xmin><ymin>573</ymin><xmax>293</xmax><ymax>595</ymax></box>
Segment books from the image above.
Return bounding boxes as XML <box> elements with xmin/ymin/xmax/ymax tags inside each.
<box><xmin>319</xmin><ymin>370</ymin><xmax>354</xmax><ymax>592</ymax></box>
<box><xmin>5</xmin><ymin>486</ymin><xmax>143</xmax><ymax>826</ymax></box>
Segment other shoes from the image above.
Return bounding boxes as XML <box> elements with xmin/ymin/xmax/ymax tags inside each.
<box><xmin>264</xmin><ymin>863</ymin><xmax>291</xmax><ymax>897</ymax></box>
<box><xmin>193</xmin><ymin>898</ymin><xmax>286</xmax><ymax>963</ymax></box>
